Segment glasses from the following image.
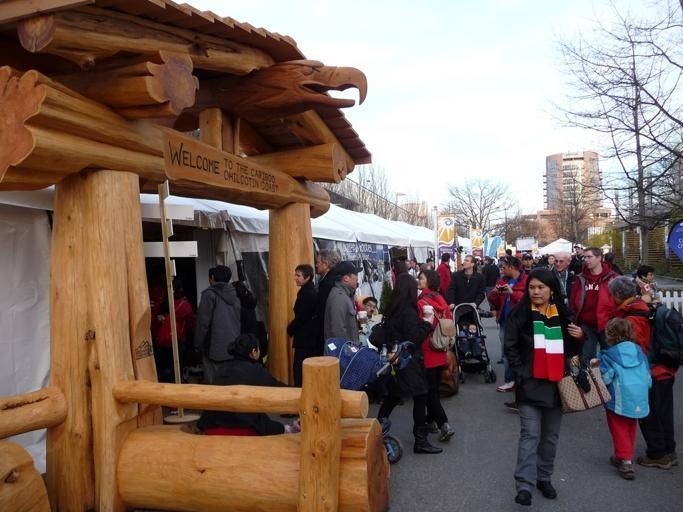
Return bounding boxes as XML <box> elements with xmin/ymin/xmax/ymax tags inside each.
<box><xmin>584</xmin><ymin>255</ymin><xmax>597</xmax><ymax>259</ymax></box>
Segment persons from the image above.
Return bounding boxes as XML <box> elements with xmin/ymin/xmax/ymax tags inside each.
<box><xmin>281</xmin><ymin>243</ymin><xmax>542</xmax><ymax>455</ymax></box>
<box><xmin>539</xmin><ymin>244</ymin><xmax>677</xmax><ymax>469</ymax></box>
<box><xmin>502</xmin><ymin>267</ymin><xmax>587</xmax><ymax>506</ymax></box>
<box><xmin>589</xmin><ymin>316</ymin><xmax>652</xmax><ymax>481</ymax></box>
<box><xmin>148</xmin><ymin>266</ymin><xmax>292</xmax><ymax>436</ymax></box>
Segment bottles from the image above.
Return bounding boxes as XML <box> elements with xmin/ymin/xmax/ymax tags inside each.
<box><xmin>379</xmin><ymin>343</ymin><xmax>388</xmax><ymax>363</ymax></box>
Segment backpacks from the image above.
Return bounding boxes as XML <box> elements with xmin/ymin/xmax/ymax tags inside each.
<box><xmin>622</xmin><ymin>301</ymin><xmax>683</xmax><ymax>367</ymax></box>
<box><xmin>422</xmin><ymin>298</ymin><xmax>457</xmax><ymax>353</ymax></box>
<box><xmin>369</xmin><ymin>300</ymin><xmax>408</xmax><ymax>353</ymax></box>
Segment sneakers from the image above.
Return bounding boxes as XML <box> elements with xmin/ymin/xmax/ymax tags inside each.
<box><xmin>504</xmin><ymin>400</ymin><xmax>520</xmax><ymax>412</ymax></box>
<box><xmin>536</xmin><ymin>480</ymin><xmax>557</xmax><ymax>498</ymax></box>
<box><xmin>514</xmin><ymin>491</ymin><xmax>532</xmax><ymax>506</ymax></box>
<box><xmin>636</xmin><ymin>451</ymin><xmax>679</xmax><ymax>470</ymax></box>
<box><xmin>439</xmin><ymin>422</ymin><xmax>456</xmax><ymax>442</ymax></box>
<box><xmin>610</xmin><ymin>455</ymin><xmax>636</xmax><ymax>480</ymax></box>
<box><xmin>496</xmin><ymin>380</ymin><xmax>517</xmax><ymax>393</ymax></box>
<box><xmin>426</xmin><ymin>420</ymin><xmax>440</xmax><ymax>433</ymax></box>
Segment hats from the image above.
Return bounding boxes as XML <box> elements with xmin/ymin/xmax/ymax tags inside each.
<box><xmin>333</xmin><ymin>259</ymin><xmax>364</xmax><ymax>277</ymax></box>
<box><xmin>522</xmin><ymin>253</ymin><xmax>534</xmax><ymax>260</ymax></box>
<box><xmin>574</xmin><ymin>243</ymin><xmax>584</xmax><ymax>249</ymax></box>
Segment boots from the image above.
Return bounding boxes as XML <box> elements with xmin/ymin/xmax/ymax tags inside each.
<box><xmin>412</xmin><ymin>423</ymin><xmax>443</xmax><ymax>454</ymax></box>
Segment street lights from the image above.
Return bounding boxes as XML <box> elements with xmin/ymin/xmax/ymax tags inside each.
<box><xmin>394</xmin><ymin>192</ymin><xmax>405</xmax><ymax>220</ymax></box>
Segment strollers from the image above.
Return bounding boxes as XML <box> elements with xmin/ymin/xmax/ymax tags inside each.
<box><xmin>452</xmin><ymin>301</ymin><xmax>498</xmax><ymax>386</ymax></box>
<box><xmin>320</xmin><ymin>338</ymin><xmax>417</xmax><ymax>464</ymax></box>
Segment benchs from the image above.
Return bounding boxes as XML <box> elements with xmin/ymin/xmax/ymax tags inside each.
<box><xmin>115</xmin><ymin>359</ymin><xmax>390</xmax><ymax>512</ymax></box>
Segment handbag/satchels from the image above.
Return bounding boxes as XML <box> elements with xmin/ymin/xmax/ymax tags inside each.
<box><xmin>557</xmin><ymin>321</ymin><xmax>612</xmax><ymax>415</ymax></box>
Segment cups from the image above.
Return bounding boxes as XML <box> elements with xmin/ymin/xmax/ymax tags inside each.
<box><xmin>423</xmin><ymin>305</ymin><xmax>434</xmax><ymax>319</ymax></box>
<box><xmin>358</xmin><ymin>311</ymin><xmax>367</xmax><ymax>329</ymax></box>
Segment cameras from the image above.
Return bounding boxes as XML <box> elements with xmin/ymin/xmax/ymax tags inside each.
<box><xmin>498</xmin><ymin>287</ymin><xmax>506</xmax><ymax>294</ymax></box>
<box><xmin>575</xmin><ymin>369</ymin><xmax>591</xmax><ymax>392</ymax></box>
